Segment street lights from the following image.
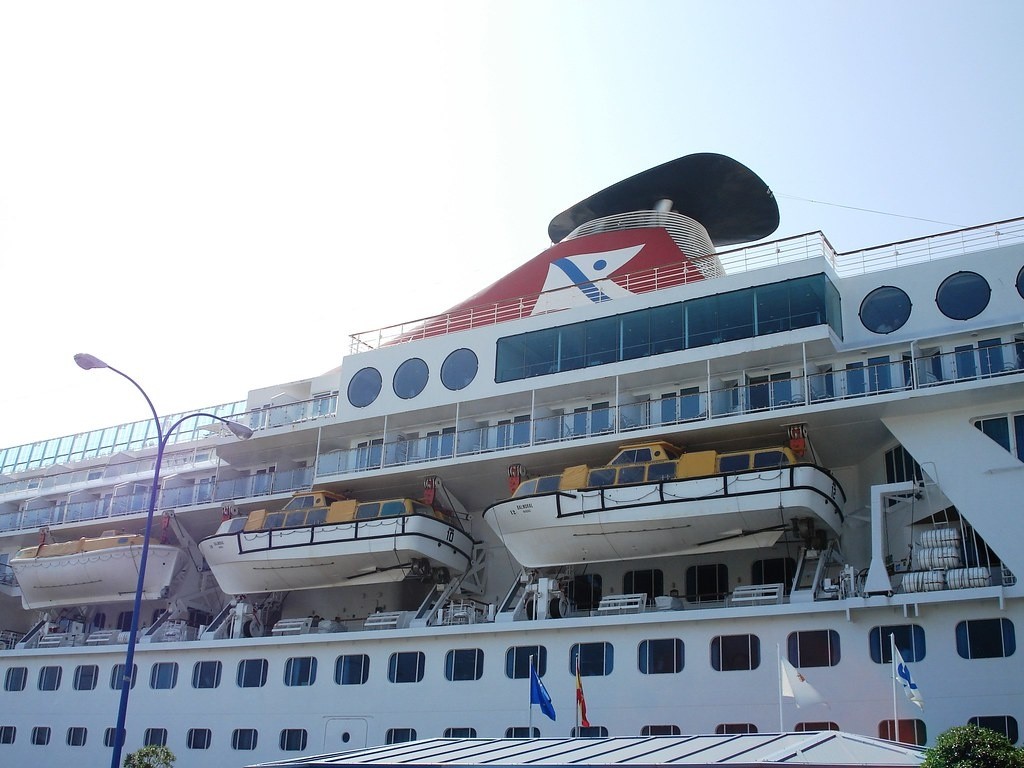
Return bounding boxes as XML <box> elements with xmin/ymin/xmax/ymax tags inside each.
<box><xmin>73</xmin><ymin>354</ymin><xmax>253</xmax><ymax>768</ymax></box>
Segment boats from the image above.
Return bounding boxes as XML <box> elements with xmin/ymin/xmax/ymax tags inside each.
<box><xmin>481</xmin><ymin>443</ymin><xmax>845</xmax><ymax>569</ymax></box>
<box><xmin>28</xmin><ymin>530</ymin><xmax>188</xmax><ymax>609</ymax></box>
<box><xmin>200</xmin><ymin>489</ymin><xmax>474</xmax><ymax>594</ymax></box>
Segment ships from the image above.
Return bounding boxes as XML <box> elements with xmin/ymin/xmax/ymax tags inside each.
<box><xmin>0</xmin><ymin>153</ymin><xmax>1024</xmax><ymax>768</ymax></box>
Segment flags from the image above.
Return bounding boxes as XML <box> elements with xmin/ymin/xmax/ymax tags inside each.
<box><xmin>576</xmin><ymin>654</ymin><xmax>590</xmax><ymax>727</ymax></box>
<box><xmin>780</xmin><ymin>653</ymin><xmax>822</xmax><ymax>708</ymax></box>
<box><xmin>530</xmin><ymin>658</ymin><xmax>556</xmax><ymax>721</ymax></box>
<box><xmin>893</xmin><ymin>644</ymin><xmax>925</xmax><ymax>712</ymax></box>
